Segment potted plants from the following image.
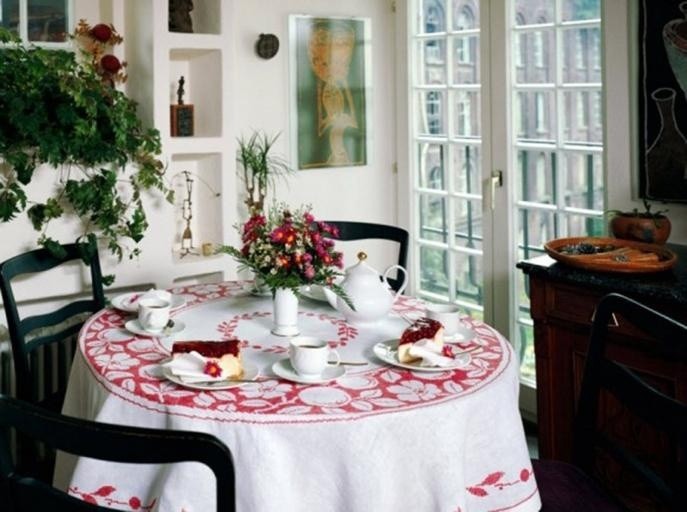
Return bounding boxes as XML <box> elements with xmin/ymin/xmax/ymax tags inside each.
<box><xmin>605</xmin><ymin>197</ymin><xmax>671</xmax><ymax>246</ymax></box>
<box><xmin>233</xmin><ymin>126</ymin><xmax>297</xmax><ymax>297</ymax></box>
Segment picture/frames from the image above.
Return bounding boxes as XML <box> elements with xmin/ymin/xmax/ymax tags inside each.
<box><xmin>625</xmin><ymin>1</ymin><xmax>686</xmax><ymax>206</ymax></box>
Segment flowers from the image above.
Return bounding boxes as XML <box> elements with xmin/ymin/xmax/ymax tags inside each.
<box><xmin>210</xmin><ymin>201</ymin><xmax>357</xmax><ymax>313</ymax></box>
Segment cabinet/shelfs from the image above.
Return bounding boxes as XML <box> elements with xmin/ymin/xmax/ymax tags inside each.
<box><xmin>521</xmin><ymin>240</ymin><xmax>687</xmax><ymax>460</ymax></box>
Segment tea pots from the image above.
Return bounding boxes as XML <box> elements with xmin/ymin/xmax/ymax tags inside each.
<box><xmin>321</xmin><ymin>252</ymin><xmax>409</xmax><ymax>323</ymax></box>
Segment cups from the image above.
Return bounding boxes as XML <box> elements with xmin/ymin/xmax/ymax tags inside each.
<box><xmin>254</xmin><ymin>274</ymin><xmax>273</xmax><ymax>294</ymax></box>
<box><xmin>425</xmin><ymin>304</ymin><xmax>460</xmax><ymax>337</ymax></box>
<box><xmin>138</xmin><ymin>298</ymin><xmax>172</xmax><ymax>333</ymax></box>
<box><xmin>290</xmin><ymin>336</ymin><xmax>342</xmax><ymax>379</ymax></box>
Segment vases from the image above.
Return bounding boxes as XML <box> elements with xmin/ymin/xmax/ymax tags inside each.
<box><xmin>271</xmin><ymin>286</ymin><xmax>301</xmax><ymax>337</ymax></box>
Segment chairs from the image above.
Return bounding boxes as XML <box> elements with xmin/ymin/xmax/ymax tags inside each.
<box><xmin>0</xmin><ymin>233</ymin><xmax>106</xmax><ymax>484</ymax></box>
<box><xmin>303</xmin><ymin>221</ymin><xmax>409</xmax><ymax>295</ymax></box>
<box><xmin>530</xmin><ymin>292</ymin><xmax>687</xmax><ymax>512</ymax></box>
<box><xmin>0</xmin><ymin>396</ymin><xmax>236</xmax><ymax>512</ymax></box>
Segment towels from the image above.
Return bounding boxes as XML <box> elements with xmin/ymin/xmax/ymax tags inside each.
<box><xmin>170</xmin><ymin>350</ymin><xmax>225</xmax><ymax>384</ymax></box>
<box><xmin>408</xmin><ymin>338</ymin><xmax>462</xmax><ymax>368</ymax></box>
<box><xmin>122</xmin><ymin>287</ymin><xmax>166</xmax><ymax>309</ymax></box>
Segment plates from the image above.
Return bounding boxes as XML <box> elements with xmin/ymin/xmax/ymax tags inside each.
<box><xmin>297</xmin><ymin>275</ymin><xmax>344</xmax><ymax>304</ymax></box>
<box><xmin>243</xmin><ymin>283</ymin><xmax>274</xmax><ymax>298</ymax></box>
<box><xmin>111</xmin><ymin>291</ymin><xmax>187</xmax><ymax>312</ymax></box>
<box><xmin>445</xmin><ymin>324</ymin><xmax>469</xmax><ymax>344</ymax></box>
<box><xmin>160</xmin><ymin>356</ymin><xmax>260</xmax><ymax>391</ymax></box>
<box><xmin>125</xmin><ymin>318</ymin><xmax>186</xmax><ymax>336</ymax></box>
<box><xmin>374</xmin><ymin>339</ymin><xmax>474</xmax><ymax>375</ymax></box>
<box><xmin>544</xmin><ymin>236</ymin><xmax>676</xmax><ymax>273</ymax></box>
<box><xmin>272</xmin><ymin>357</ymin><xmax>345</xmax><ymax>385</ymax></box>
